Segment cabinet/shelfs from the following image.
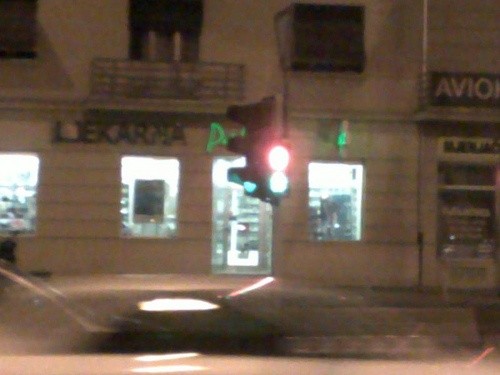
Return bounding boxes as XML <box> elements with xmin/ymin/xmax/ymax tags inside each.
<box><xmin>420</xmin><ymin>136</ymin><xmax>500</xmax><ymax>288</ymax></box>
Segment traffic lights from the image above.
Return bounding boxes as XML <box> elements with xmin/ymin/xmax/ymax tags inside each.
<box><xmin>227</xmin><ymin>95</ymin><xmax>279</xmax><ymax>205</ymax></box>
<box><xmin>266</xmin><ymin>141</ymin><xmax>292</xmax><ymax>198</ymax></box>
